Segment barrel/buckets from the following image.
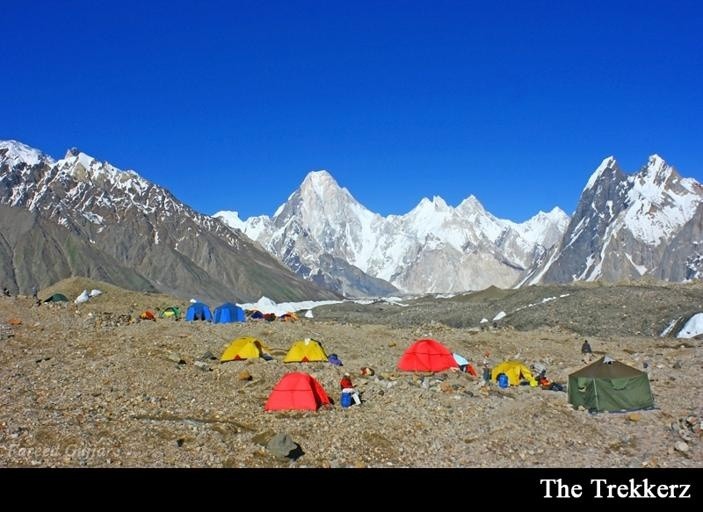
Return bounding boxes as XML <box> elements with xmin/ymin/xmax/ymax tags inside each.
<box><xmin>498</xmin><ymin>374</ymin><xmax>508</xmax><ymax>388</ymax></box>
<box><xmin>341</xmin><ymin>393</ymin><xmax>352</xmax><ymax>408</ymax></box>
<box><xmin>482</xmin><ymin>368</ymin><xmax>493</xmax><ymax>379</ymax></box>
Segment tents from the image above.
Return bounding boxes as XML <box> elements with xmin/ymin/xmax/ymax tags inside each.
<box><xmin>2</xmin><ymin>286</ymin><xmax>703</xmax><ymax>416</ymax></box>
